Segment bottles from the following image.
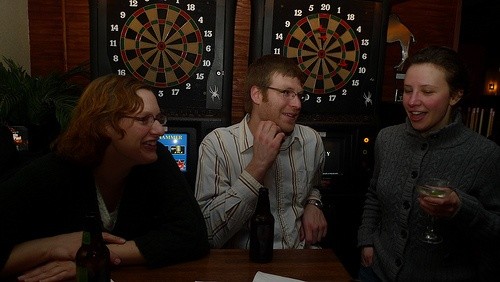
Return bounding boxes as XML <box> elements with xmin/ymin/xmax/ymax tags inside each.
<box><xmin>249</xmin><ymin>188</ymin><xmax>275</xmax><ymax>263</ymax></box>
<box><xmin>76</xmin><ymin>211</ymin><xmax>110</xmax><ymax>282</ymax></box>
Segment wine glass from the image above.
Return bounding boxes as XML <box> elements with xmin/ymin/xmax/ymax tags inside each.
<box><xmin>414</xmin><ymin>178</ymin><xmax>452</xmax><ymax>244</ymax></box>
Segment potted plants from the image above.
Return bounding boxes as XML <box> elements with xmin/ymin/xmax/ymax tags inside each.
<box><xmin>0</xmin><ymin>54</ymin><xmax>90</xmax><ymax>152</ymax></box>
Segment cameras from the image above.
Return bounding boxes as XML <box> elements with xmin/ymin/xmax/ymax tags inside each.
<box><xmin>158</xmin><ymin>126</ymin><xmax>198</xmax><ymax>179</ymax></box>
<box><xmin>316</xmin><ymin>131</ymin><xmax>357</xmax><ymax>184</ymax></box>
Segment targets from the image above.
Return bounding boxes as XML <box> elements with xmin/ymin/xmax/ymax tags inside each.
<box><xmin>88</xmin><ymin>0</ymin><xmax>238</xmax><ymax>126</ymax></box>
<box><xmin>263</xmin><ymin>0</ymin><xmax>391</xmax><ymax>126</ymax></box>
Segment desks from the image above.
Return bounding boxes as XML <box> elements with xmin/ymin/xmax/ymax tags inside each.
<box><xmin>0</xmin><ymin>247</ymin><xmax>356</xmax><ymax>281</ymax></box>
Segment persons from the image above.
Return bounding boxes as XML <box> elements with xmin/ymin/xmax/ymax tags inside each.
<box><xmin>357</xmin><ymin>45</ymin><xmax>500</xmax><ymax>282</ymax></box>
<box><xmin>1</xmin><ymin>74</ymin><xmax>208</xmax><ymax>282</ymax></box>
<box><xmin>194</xmin><ymin>55</ymin><xmax>327</xmax><ymax>250</ymax></box>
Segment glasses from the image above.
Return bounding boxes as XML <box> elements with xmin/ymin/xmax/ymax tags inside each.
<box><xmin>265</xmin><ymin>85</ymin><xmax>306</xmax><ymax>103</ymax></box>
<box><xmin>121</xmin><ymin>112</ymin><xmax>167</xmax><ymax>126</ymax></box>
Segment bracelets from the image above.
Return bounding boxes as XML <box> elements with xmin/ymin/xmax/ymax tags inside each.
<box><xmin>306</xmin><ymin>199</ymin><xmax>321</xmax><ymax>209</ymax></box>
